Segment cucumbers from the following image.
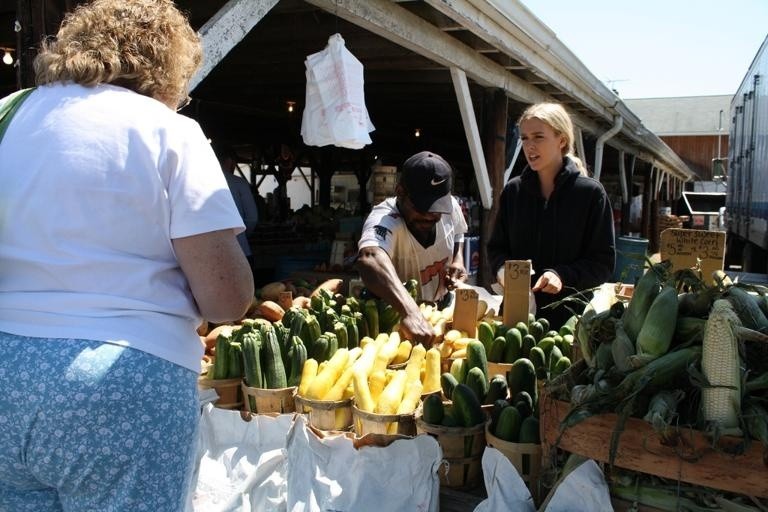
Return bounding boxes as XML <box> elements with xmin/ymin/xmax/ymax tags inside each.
<box><xmin>213</xmin><ymin>286</ymin><xmax>583</xmax><ymax>445</ymax></box>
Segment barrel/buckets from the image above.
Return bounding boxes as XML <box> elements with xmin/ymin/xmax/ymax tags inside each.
<box><xmin>612</xmin><ymin>235</ymin><xmax>649</xmax><ymax>284</ymax></box>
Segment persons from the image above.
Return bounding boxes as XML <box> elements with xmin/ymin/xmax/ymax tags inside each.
<box><xmin>215</xmin><ymin>148</ymin><xmax>259</xmax><ymax>270</ymax></box>
<box><xmin>0</xmin><ymin>0</ymin><xmax>254</xmax><ymax>512</ymax></box>
<box><xmin>358</xmin><ymin>151</ymin><xmax>468</xmax><ymax>347</ymax></box>
<box><xmin>488</xmin><ymin>101</ymin><xmax>616</xmax><ymax>330</ymax></box>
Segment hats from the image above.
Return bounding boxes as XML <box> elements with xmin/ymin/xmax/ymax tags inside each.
<box><xmin>400</xmin><ymin>152</ymin><xmax>454</xmax><ymax>215</ymax></box>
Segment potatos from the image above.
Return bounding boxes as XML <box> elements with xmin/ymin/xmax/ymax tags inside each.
<box><xmin>195</xmin><ymin>278</ymin><xmax>344</xmax><ymax>365</ymax></box>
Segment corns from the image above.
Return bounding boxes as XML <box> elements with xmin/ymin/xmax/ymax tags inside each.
<box><xmin>550</xmin><ymin>259</ymin><xmax>768</xmax><ymax>512</ymax></box>
